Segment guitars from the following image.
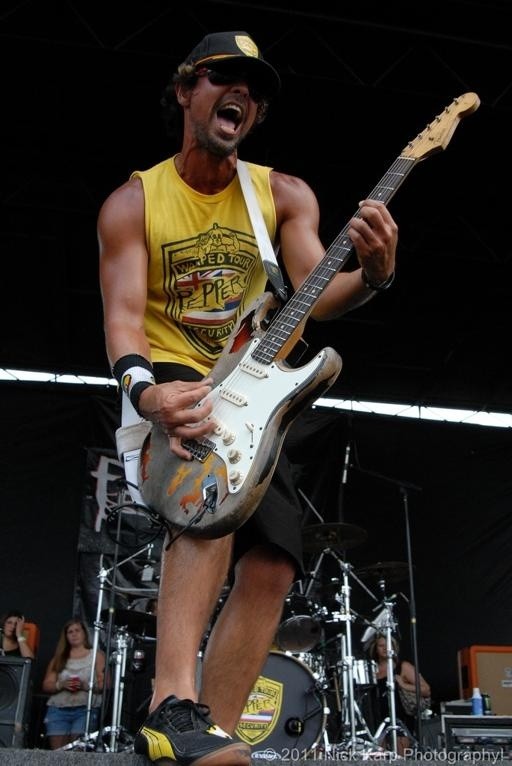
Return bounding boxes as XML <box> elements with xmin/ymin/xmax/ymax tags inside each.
<box><xmin>137</xmin><ymin>92</ymin><xmax>481</xmax><ymax>540</ymax></box>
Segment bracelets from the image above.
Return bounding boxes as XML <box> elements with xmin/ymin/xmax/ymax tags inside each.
<box><xmin>111</xmin><ymin>351</ymin><xmax>159</xmax><ymax>421</ymax></box>
<box><xmin>359</xmin><ymin>265</ymin><xmax>397</xmax><ymax>292</ymax></box>
<box><xmin>85</xmin><ymin>683</ymin><xmax>92</xmax><ymax>693</ymax></box>
<box><xmin>55</xmin><ymin>680</ymin><xmax>62</xmax><ymax>692</ymax></box>
<box><xmin>17</xmin><ymin>634</ymin><xmax>26</xmax><ymax>642</ymax></box>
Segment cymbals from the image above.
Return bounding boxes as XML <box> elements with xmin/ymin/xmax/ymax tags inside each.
<box><xmin>356</xmin><ymin>562</ymin><xmax>414</xmax><ymax>576</ymax></box>
<box><xmin>301</xmin><ymin>522</ymin><xmax>362</xmax><ymax>550</ymax></box>
<box><xmin>101</xmin><ymin>608</ymin><xmax>156</xmax><ymax>638</ymax></box>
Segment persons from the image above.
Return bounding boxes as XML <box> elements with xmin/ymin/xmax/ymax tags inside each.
<box><xmin>0</xmin><ymin>606</ymin><xmax>37</xmax><ymax>664</ymax></box>
<box><xmin>369</xmin><ymin>630</ymin><xmax>433</xmax><ymax>761</ymax></box>
<box><xmin>94</xmin><ymin>25</ymin><xmax>405</xmax><ymax>765</ymax></box>
<box><xmin>40</xmin><ymin>615</ymin><xmax>108</xmax><ymax>750</ymax></box>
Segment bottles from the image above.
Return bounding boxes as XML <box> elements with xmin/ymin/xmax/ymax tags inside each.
<box><xmin>471</xmin><ymin>685</ymin><xmax>492</xmax><ymax>716</ymax></box>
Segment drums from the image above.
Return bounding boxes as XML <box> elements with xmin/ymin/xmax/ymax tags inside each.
<box><xmin>232</xmin><ymin>650</ymin><xmax>327</xmax><ymax>760</ymax></box>
<box><xmin>337</xmin><ymin>656</ymin><xmax>377</xmax><ymax>692</ymax></box>
<box><xmin>275</xmin><ymin>592</ymin><xmax>321</xmax><ymax>655</ymax></box>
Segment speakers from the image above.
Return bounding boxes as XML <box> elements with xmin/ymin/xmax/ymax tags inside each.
<box><xmin>0</xmin><ymin>655</ymin><xmax>33</xmax><ymax>749</ymax></box>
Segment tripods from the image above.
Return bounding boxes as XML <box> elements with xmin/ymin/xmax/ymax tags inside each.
<box><xmin>326</xmin><ymin>635</ymin><xmax>422</xmax><ymax>762</ymax></box>
<box><xmin>52</xmin><ymin>610</ymin><xmax>136</xmax><ymax>753</ymax></box>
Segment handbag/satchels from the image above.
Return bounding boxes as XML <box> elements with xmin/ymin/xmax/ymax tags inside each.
<box><xmin>397</xmin><ymin>661</ymin><xmax>432</xmax><ymax>716</ymax></box>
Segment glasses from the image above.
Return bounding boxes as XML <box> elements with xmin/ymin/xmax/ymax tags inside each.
<box><xmin>184</xmin><ymin>66</ymin><xmax>270</xmax><ymax>104</ymax></box>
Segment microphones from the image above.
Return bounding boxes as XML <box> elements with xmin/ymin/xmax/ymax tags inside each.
<box><xmin>284</xmin><ymin>716</ymin><xmax>304</xmax><ymax>738</ymax></box>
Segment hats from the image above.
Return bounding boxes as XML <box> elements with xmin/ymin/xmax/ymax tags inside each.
<box><xmin>181</xmin><ymin>29</ymin><xmax>282</xmax><ymax>103</ymax></box>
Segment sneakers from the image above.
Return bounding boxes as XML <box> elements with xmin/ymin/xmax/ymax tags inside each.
<box><xmin>134</xmin><ymin>697</ymin><xmax>252</xmax><ymax>766</ymax></box>
